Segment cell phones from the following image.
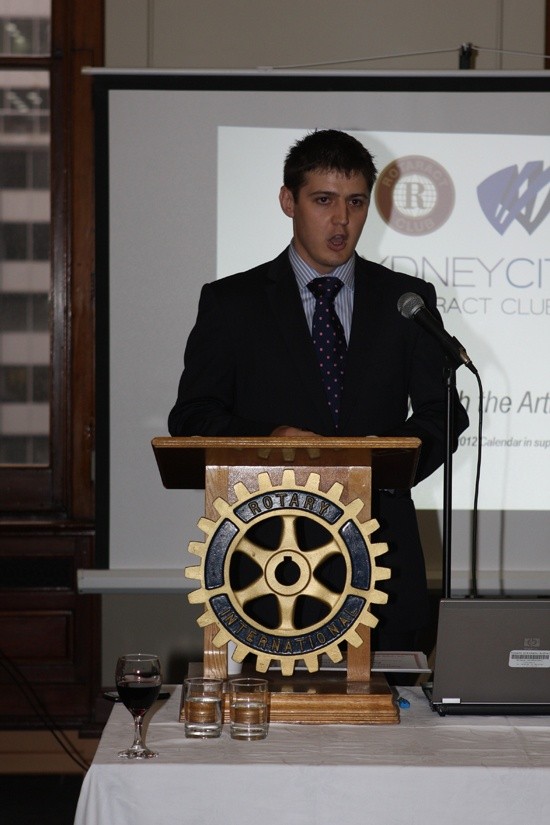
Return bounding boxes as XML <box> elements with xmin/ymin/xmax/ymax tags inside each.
<box><xmin>101</xmin><ymin>688</ymin><xmax>170</xmax><ymax>702</ymax></box>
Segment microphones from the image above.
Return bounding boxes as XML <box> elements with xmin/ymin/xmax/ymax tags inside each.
<box><xmin>396</xmin><ymin>292</ymin><xmax>479</xmax><ymax>374</ymax></box>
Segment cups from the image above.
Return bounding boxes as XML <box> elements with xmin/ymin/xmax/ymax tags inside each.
<box><xmin>182</xmin><ymin>677</ymin><xmax>225</xmax><ymax>739</ymax></box>
<box><xmin>228</xmin><ymin>678</ymin><xmax>270</xmax><ymax>741</ymax></box>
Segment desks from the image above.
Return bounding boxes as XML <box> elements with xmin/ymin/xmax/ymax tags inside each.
<box><xmin>74</xmin><ymin>686</ymin><xmax>550</xmax><ymax>825</ymax></box>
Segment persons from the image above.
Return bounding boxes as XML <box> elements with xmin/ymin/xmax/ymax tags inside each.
<box><xmin>169</xmin><ymin>128</ymin><xmax>470</xmax><ymax>685</ymax></box>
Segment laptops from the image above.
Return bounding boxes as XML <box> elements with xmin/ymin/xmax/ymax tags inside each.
<box><xmin>421</xmin><ymin>600</ymin><xmax>550</xmax><ymax>716</ymax></box>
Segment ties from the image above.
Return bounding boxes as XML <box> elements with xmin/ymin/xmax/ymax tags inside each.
<box><xmin>306</xmin><ymin>277</ymin><xmax>347</xmax><ymax>431</ymax></box>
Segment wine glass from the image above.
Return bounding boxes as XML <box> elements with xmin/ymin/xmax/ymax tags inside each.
<box><xmin>115</xmin><ymin>652</ymin><xmax>161</xmax><ymax>760</ymax></box>
